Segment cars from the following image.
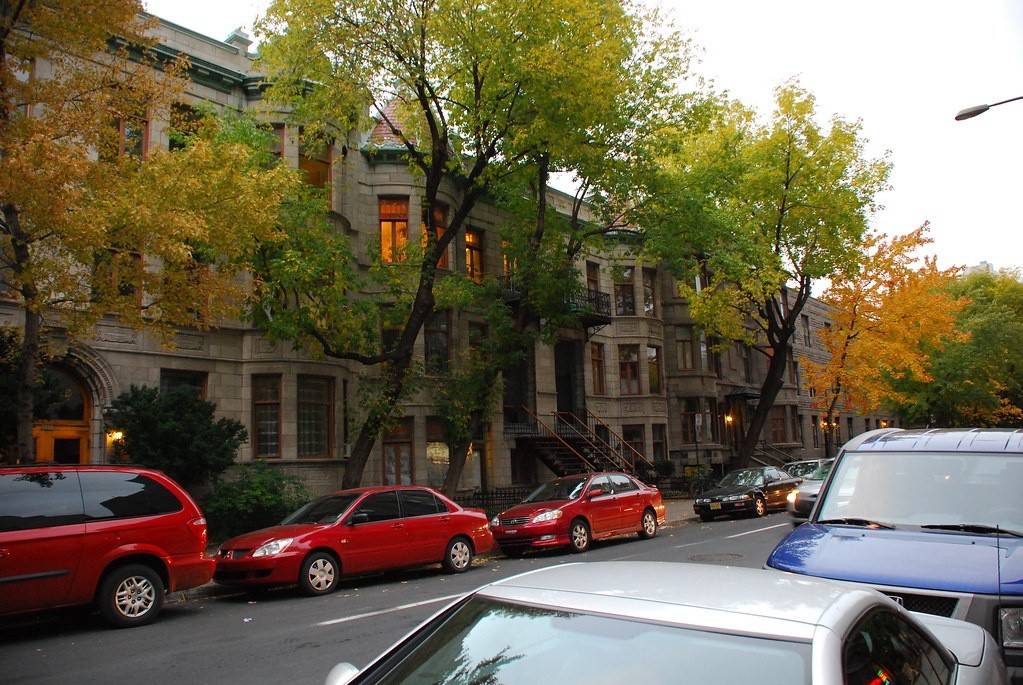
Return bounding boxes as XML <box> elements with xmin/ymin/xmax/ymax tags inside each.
<box><xmin>785</xmin><ymin>456</ymin><xmax>869</xmax><ymax>526</ymax></box>
<box><xmin>324</xmin><ymin>558</ymin><xmax>1014</xmax><ymax>685</ymax></box>
<box><xmin>694</xmin><ymin>466</ymin><xmax>803</xmax><ymax>522</ymax></box>
<box><xmin>210</xmin><ymin>484</ymin><xmax>495</xmax><ymax>596</ymax></box>
<box><xmin>488</xmin><ymin>470</ymin><xmax>667</xmax><ymax>557</ymax></box>
<box><xmin>780</xmin><ymin>459</ymin><xmax>827</xmax><ymax>478</ymax></box>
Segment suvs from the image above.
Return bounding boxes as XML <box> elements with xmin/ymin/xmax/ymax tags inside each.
<box><xmin>761</xmin><ymin>423</ymin><xmax>1023</xmax><ymax>685</ymax></box>
<box><xmin>0</xmin><ymin>462</ymin><xmax>219</xmax><ymax>630</ymax></box>
